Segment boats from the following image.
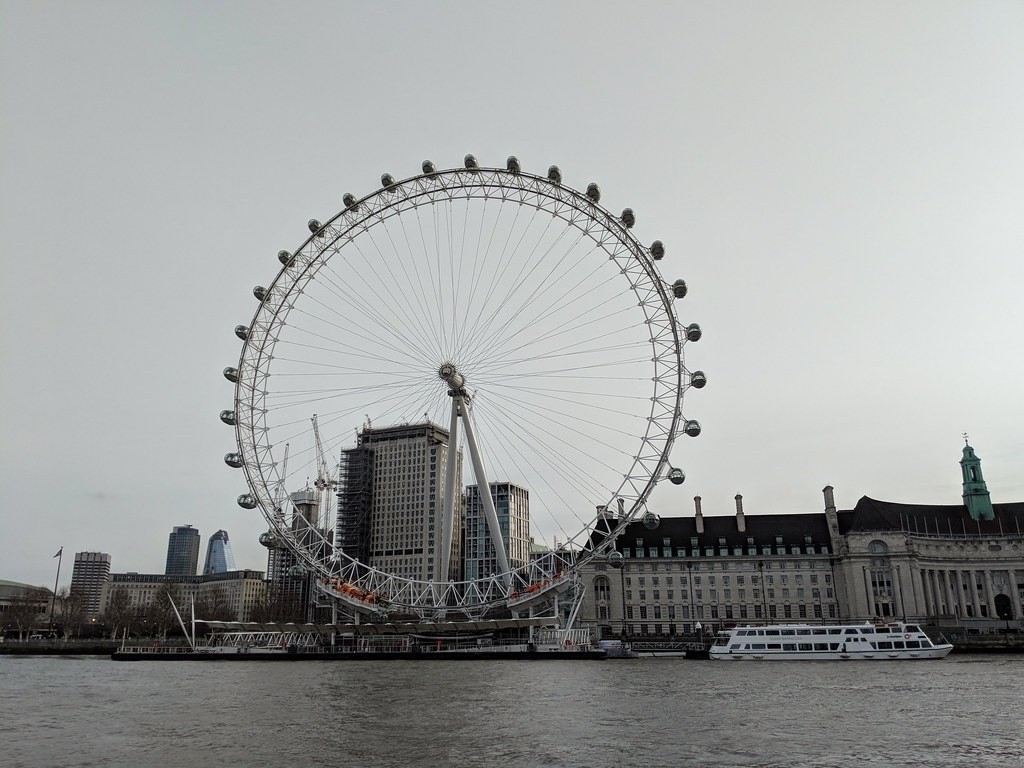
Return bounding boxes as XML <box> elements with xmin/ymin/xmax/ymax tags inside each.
<box><xmin>709</xmin><ymin>620</ymin><xmax>953</xmax><ymax>660</ymax></box>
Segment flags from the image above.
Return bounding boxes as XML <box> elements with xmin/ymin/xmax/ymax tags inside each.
<box><xmin>54</xmin><ymin>550</ymin><xmax>60</xmax><ymax>557</ymax></box>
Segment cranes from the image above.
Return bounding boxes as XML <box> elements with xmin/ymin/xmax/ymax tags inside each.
<box><xmin>306</xmin><ymin>412</ymin><xmax>338</xmax><ymax>625</ymax></box>
<box><xmin>264</xmin><ymin>442</ymin><xmax>289</xmax><ymax>622</ymax></box>
